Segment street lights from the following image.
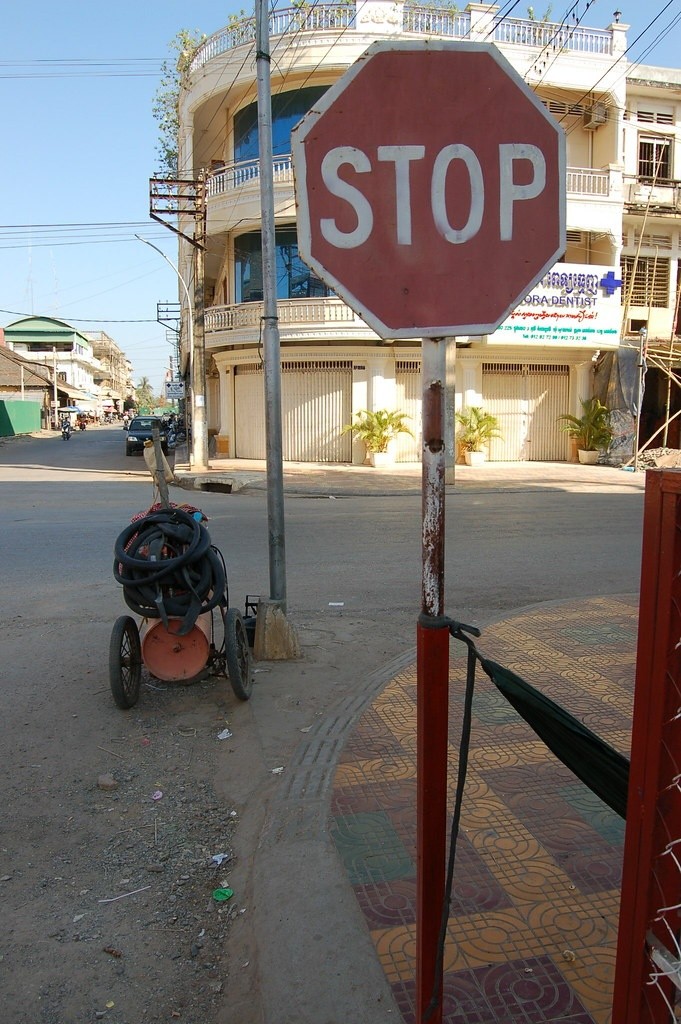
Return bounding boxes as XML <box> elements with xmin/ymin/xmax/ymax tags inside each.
<box><xmin>133</xmin><ymin>233</ymin><xmax>195</xmax><ymax>424</ymax></box>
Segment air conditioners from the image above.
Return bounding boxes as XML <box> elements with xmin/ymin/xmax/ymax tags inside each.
<box><xmin>582</xmin><ymin>104</ymin><xmax>605</xmax><ymax>130</ymax></box>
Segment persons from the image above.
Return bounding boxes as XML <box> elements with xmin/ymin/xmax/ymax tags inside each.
<box><xmin>79</xmin><ymin>417</ymin><xmax>87</xmax><ymax>431</ymax></box>
<box><xmin>62</xmin><ymin>417</ymin><xmax>70</xmax><ymax>438</ymax></box>
<box><xmin>122</xmin><ymin>413</ymin><xmax>129</xmax><ymax>425</ymax></box>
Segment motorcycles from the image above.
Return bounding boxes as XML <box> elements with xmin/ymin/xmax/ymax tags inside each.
<box><xmin>80</xmin><ymin>421</ymin><xmax>86</xmax><ymax>431</ymax></box>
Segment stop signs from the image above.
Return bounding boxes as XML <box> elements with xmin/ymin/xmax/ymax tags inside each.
<box><xmin>293</xmin><ymin>40</ymin><xmax>567</xmax><ymax>343</ymax></box>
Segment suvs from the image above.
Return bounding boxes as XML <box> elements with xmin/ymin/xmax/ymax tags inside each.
<box><xmin>123</xmin><ymin>416</ymin><xmax>168</xmax><ymax>456</ymax></box>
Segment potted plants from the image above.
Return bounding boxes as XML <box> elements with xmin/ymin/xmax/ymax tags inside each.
<box><xmin>339</xmin><ymin>408</ymin><xmax>416</xmax><ymax>467</ymax></box>
<box><xmin>454</xmin><ymin>406</ymin><xmax>506</xmax><ymax>466</ymax></box>
<box><xmin>554</xmin><ymin>394</ymin><xmax>616</xmax><ymax>465</ymax></box>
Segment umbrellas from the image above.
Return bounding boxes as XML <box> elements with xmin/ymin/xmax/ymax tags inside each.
<box><xmin>53</xmin><ymin>405</ymin><xmax>79</xmax><ymax>426</ymax></box>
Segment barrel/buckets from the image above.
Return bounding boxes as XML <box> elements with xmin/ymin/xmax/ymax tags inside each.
<box><xmin>142</xmin><ymin>589</ymin><xmax>213</xmax><ymax>681</ymax></box>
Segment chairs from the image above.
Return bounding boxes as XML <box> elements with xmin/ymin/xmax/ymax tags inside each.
<box><xmin>144</xmin><ymin>425</ymin><xmax>151</xmax><ymax>428</ymax></box>
<box><xmin>133</xmin><ymin>421</ymin><xmax>143</xmax><ymax>430</ymax></box>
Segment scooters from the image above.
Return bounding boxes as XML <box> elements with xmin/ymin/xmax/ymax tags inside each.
<box><xmin>61</xmin><ymin>423</ymin><xmax>70</xmax><ymax>442</ymax></box>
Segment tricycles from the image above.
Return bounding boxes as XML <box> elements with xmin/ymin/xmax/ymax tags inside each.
<box><xmin>109</xmin><ymin>428</ymin><xmax>253</xmax><ymax>710</ymax></box>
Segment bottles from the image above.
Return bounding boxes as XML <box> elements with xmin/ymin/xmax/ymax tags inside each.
<box><xmin>623</xmin><ymin>467</ymin><xmax>634</xmax><ymax>471</ymax></box>
